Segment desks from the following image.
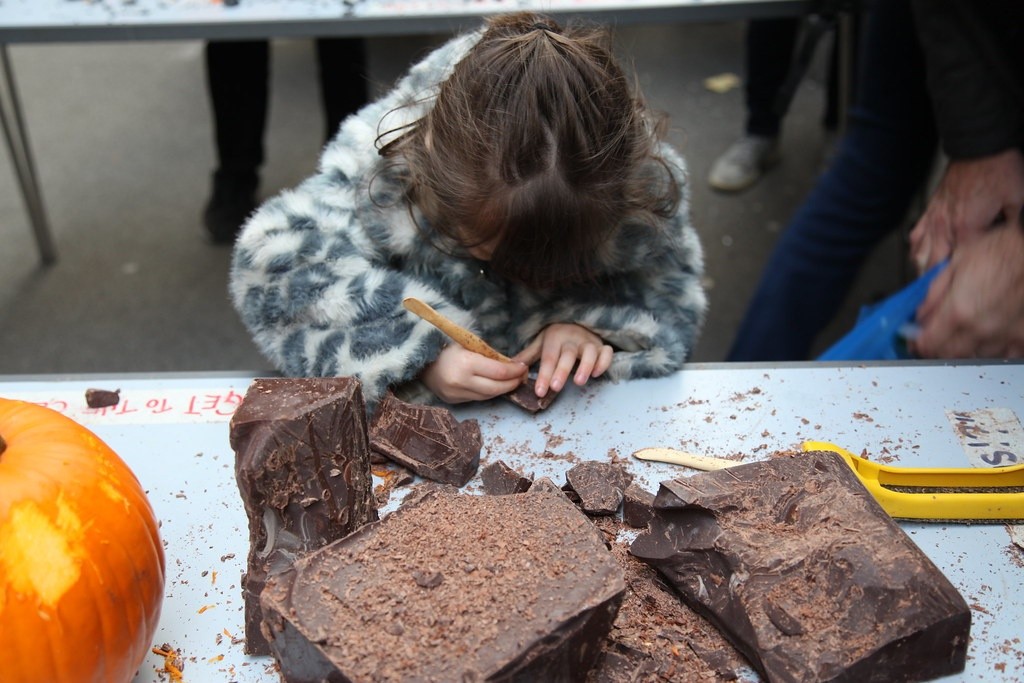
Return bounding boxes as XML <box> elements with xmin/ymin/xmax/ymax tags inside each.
<box><xmin>0</xmin><ymin>359</ymin><xmax>1023</xmax><ymax>682</ymax></box>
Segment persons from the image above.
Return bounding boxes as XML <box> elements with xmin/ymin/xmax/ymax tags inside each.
<box><xmin>227</xmin><ymin>15</ymin><xmax>711</xmax><ymax>403</ymax></box>
<box><xmin>712</xmin><ymin>7</ymin><xmax>833</xmax><ymax>190</ymax></box>
<box><xmin>729</xmin><ymin>0</ymin><xmax>1023</xmax><ymax>362</ymax></box>
<box><xmin>201</xmin><ymin>38</ymin><xmax>372</xmax><ymax>248</ymax></box>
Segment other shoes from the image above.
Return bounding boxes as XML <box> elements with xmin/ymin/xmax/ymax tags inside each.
<box><xmin>204</xmin><ymin>165</ymin><xmax>259</xmax><ymax>240</ymax></box>
<box><xmin>711</xmin><ymin>140</ymin><xmax>781</xmax><ymax>194</ymax></box>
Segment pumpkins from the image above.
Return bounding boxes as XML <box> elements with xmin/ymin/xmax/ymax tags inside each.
<box><xmin>0</xmin><ymin>397</ymin><xmax>166</xmax><ymax>683</ymax></box>
